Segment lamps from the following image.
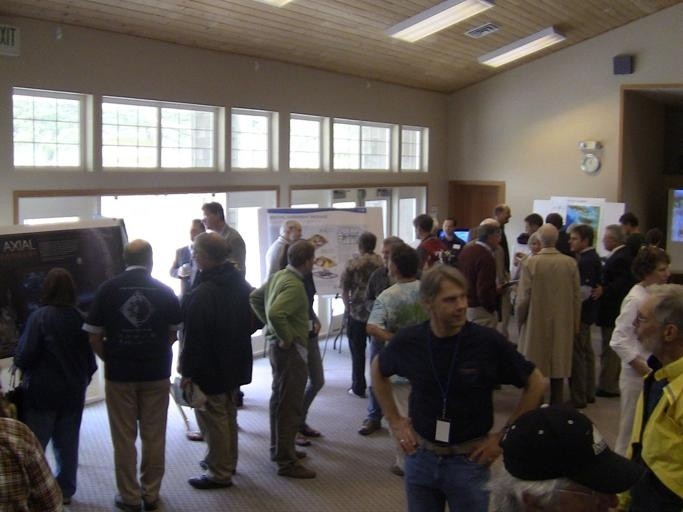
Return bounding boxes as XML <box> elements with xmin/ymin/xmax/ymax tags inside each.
<box><xmin>478</xmin><ymin>27</ymin><xmax>566</xmax><ymax>69</ymax></box>
<box><xmin>386</xmin><ymin>1</ymin><xmax>494</xmax><ymax>45</ymax></box>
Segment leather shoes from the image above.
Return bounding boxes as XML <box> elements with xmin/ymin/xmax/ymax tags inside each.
<box><xmin>142</xmin><ymin>498</ymin><xmax>159</xmax><ymax>510</ymax></box>
<box><xmin>115</xmin><ymin>494</ymin><xmax>142</xmax><ymax>511</ymax></box>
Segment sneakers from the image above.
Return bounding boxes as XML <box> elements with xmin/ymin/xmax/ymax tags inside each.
<box><xmin>358</xmin><ymin>417</ymin><xmax>379</xmax><ymax>436</ymax></box>
<box><xmin>188</xmin><ymin>473</ymin><xmax>233</xmax><ymax>490</ymax></box>
<box><xmin>271</xmin><ymin>447</ymin><xmax>305</xmax><ymax>460</ymax></box>
<box><xmin>306</xmin><ymin>426</ymin><xmax>322</xmax><ymax>439</ymax></box>
<box><xmin>294</xmin><ymin>433</ymin><xmax>309</xmax><ymax>446</ymax></box>
<box><xmin>277</xmin><ymin>462</ymin><xmax>316</xmax><ymax>478</ymax></box>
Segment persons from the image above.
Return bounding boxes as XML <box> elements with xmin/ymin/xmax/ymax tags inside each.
<box><xmin>199</xmin><ymin>202</ymin><xmax>246</xmax><ymax>282</ymax></box>
<box><xmin>263</xmin><ymin>219</ymin><xmax>302</xmax><ymax>280</ymax></box>
<box><xmin>294</xmin><ymin>267</ymin><xmax>324</xmax><ymax>446</ymax></box>
<box><xmin>337</xmin><ymin>204</ymin><xmax>673</xmax><ymax>461</ymax></box>
<box><xmin>85</xmin><ymin>239</ymin><xmax>183</xmax><ymax>512</ymax></box>
<box><xmin>169</xmin><ymin>218</ymin><xmax>206</xmax><ymax>296</ymax></box>
<box><xmin>608</xmin><ymin>284</ymin><xmax>682</xmax><ymax>511</ymax></box>
<box><xmin>15</xmin><ymin>267</ymin><xmax>97</xmax><ymax>504</ymax></box>
<box><xmin>0</xmin><ymin>381</ymin><xmax>65</xmax><ymax>512</ymax></box>
<box><xmin>476</xmin><ymin>402</ymin><xmax>648</xmax><ymax>511</ymax></box>
<box><xmin>248</xmin><ymin>240</ymin><xmax>315</xmax><ymax>479</ymax></box>
<box><xmin>177</xmin><ymin>230</ymin><xmax>251</xmax><ymax>489</ymax></box>
<box><xmin>364</xmin><ymin>246</ymin><xmax>450</xmax><ymax>475</ymax></box>
<box><xmin>369</xmin><ymin>265</ymin><xmax>549</xmax><ymax>511</ymax></box>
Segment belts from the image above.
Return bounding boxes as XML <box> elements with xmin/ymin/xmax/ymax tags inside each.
<box><xmin>414</xmin><ymin>434</ymin><xmax>481</xmax><ymax>456</ymax></box>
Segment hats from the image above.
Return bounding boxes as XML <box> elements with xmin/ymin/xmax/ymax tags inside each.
<box><xmin>499</xmin><ymin>404</ymin><xmax>646</xmax><ymax>493</ymax></box>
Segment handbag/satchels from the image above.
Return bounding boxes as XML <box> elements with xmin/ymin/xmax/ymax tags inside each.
<box><xmin>4</xmin><ymin>366</ymin><xmax>24</xmax><ymax>420</ymax></box>
